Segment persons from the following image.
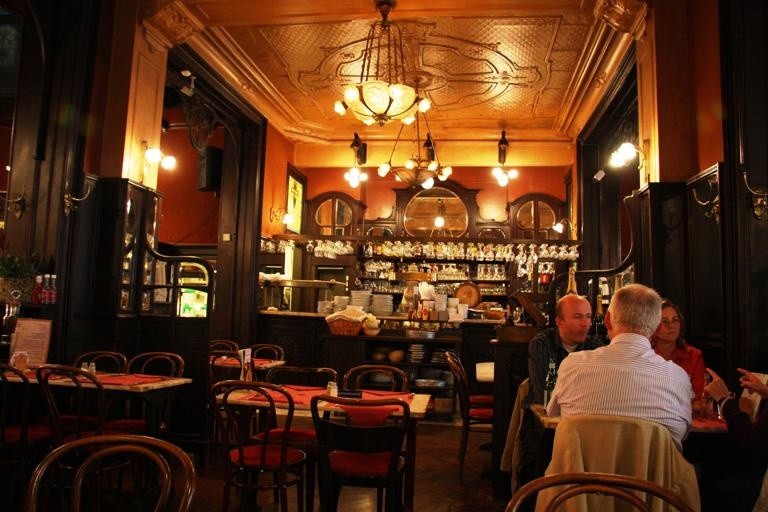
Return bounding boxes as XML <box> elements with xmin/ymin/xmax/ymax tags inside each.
<box><xmin>701</xmin><ymin>367</ymin><xmax>768</xmax><ymax>512</ymax></box>
<box><xmin>650</xmin><ymin>298</ymin><xmax>707</xmax><ymax>419</ymax></box>
<box><xmin>546</xmin><ymin>284</ymin><xmax>693</xmax><ymax>456</ymax></box>
<box><xmin>519</xmin><ymin>293</ymin><xmax>605</xmax><ymax>486</ymax></box>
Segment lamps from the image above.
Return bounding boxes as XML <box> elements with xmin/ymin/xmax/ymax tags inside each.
<box><xmin>334</xmin><ymin>0</ymin><xmax>650</xmax><ymax>192</ymax></box>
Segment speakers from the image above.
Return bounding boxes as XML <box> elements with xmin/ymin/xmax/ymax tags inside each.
<box><xmin>197</xmin><ymin>146</ymin><xmax>224</xmax><ymax>192</ymax></box>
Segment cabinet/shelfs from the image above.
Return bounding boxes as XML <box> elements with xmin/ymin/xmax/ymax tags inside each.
<box><xmin>362</xmin><ymin>256</ymin><xmax>509</xmax><ymax>302</ymax></box>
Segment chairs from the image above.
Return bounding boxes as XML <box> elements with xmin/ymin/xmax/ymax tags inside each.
<box><xmin>0</xmin><ymin>340</ymin><xmax>432</xmax><ymax>511</ymax></box>
<box><xmin>444</xmin><ymin>351</ymin><xmax>556</xmax><ymax>479</ymax></box>
<box><xmin>506</xmin><ymin>472</ymin><xmax>691</xmax><ymax>509</ymax></box>
<box><xmin>560</xmin><ymin>414</ymin><xmax>683</xmax><ymax>511</ymax></box>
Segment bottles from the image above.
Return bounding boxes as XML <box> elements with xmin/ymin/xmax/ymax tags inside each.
<box><xmin>32</xmin><ymin>274</ymin><xmax>57</xmax><ymax>305</ymax></box>
<box><xmin>543</xmin><ymin>357</ymin><xmax>558</xmax><ymax>412</ymax></box>
<box><xmin>80</xmin><ymin>362</ymin><xmax>96</xmax><ymax>377</ymax></box>
<box><xmin>593</xmin><ymin>294</ymin><xmax>605</xmax><ymax>339</ymax></box>
<box><xmin>326</xmin><ymin>381</ymin><xmax>339</xmax><ymax>398</ymax></box>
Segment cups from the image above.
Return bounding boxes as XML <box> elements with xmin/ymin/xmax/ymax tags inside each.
<box><xmin>372</xmin><ymin>346</ymin><xmax>406</xmax><ymax>362</ymax></box>
<box><xmin>443</xmin><ymin>371</ymin><xmax>454</xmax><ymax>388</ymax></box>
<box><xmin>407</xmin><ymin>281</ymin><xmax>471</xmax><ymax>323</ymax></box>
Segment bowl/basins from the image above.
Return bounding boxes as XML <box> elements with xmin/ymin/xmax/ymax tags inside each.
<box><xmin>363</xmin><ymin>327</ymin><xmax>380</xmax><ymax>336</ymax></box>
<box><xmin>405</xmin><ymin>330</ymin><xmax>436</xmax><ymax>340</ymax></box>
<box><xmin>416</xmin><ymin>379</ymin><xmax>446</xmax><ymax>386</ymax></box>
<box><xmin>484</xmin><ymin>309</ymin><xmax>506</xmax><ymax>320</ymax></box>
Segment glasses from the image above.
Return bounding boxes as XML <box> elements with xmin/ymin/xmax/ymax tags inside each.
<box><xmin>661</xmin><ymin>316</ymin><xmax>682</xmax><ymax>328</ymax></box>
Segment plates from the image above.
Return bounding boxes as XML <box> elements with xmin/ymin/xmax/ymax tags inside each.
<box><xmin>431</xmin><ymin>348</ymin><xmax>454</xmax><ymax>364</ymax></box>
<box><xmin>318</xmin><ymin>288</ymin><xmax>395</xmax><ymax>316</ymax></box>
<box><xmin>409</xmin><ymin>344</ymin><xmax>425</xmax><ymax>363</ymax></box>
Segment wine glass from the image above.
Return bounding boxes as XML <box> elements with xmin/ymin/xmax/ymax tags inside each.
<box><xmin>303</xmin><ymin>237</ymin><xmax>353</xmax><ymax>257</ymax></box>
<box><xmin>364</xmin><ymin>239</ymin><xmax>581</xmax><ymax>296</ymax></box>
